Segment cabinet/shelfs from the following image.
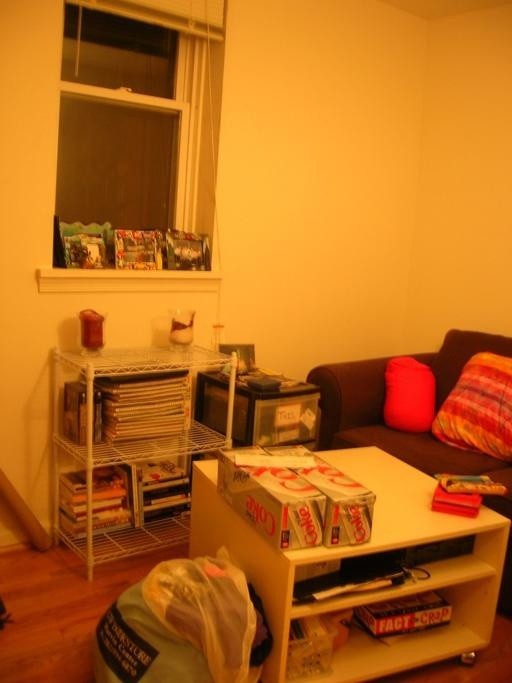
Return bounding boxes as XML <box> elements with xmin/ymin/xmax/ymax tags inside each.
<box><xmin>189</xmin><ymin>446</ymin><xmax>512</xmax><ymax>683</ymax></box>
<box><xmin>51</xmin><ymin>344</ymin><xmax>239</xmax><ymax>583</ymax></box>
<box><xmin>194</xmin><ymin>367</ymin><xmax>323</xmax><ymax>462</ymax></box>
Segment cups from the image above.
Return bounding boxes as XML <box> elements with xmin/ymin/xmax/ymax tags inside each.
<box><xmin>77</xmin><ymin>309</ymin><xmax>106</xmax><ymax>359</ymax></box>
<box><xmin>169</xmin><ymin>307</ymin><xmax>195</xmax><ymax>351</ymax></box>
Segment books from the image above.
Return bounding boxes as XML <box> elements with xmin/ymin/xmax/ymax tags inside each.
<box><xmin>429</xmin><ymin>498</ymin><xmax>479</xmax><ymax>517</ymax></box>
<box><xmin>352</xmin><ymin>590</ymin><xmax>452</xmax><ymax>639</ymax></box>
<box><xmin>432</xmin><ymin>483</ymin><xmax>484</xmax><ymax>510</ymax></box>
<box><xmin>57</xmin><ymin>368</ymin><xmax>193</xmax><ymax>542</ymax></box>
<box><xmin>432</xmin><ymin>470</ymin><xmax>507</xmax><ymax>498</ymax></box>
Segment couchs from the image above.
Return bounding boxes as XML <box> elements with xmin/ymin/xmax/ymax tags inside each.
<box><xmin>305</xmin><ymin>329</ymin><xmax>512</xmax><ymax>621</ymax></box>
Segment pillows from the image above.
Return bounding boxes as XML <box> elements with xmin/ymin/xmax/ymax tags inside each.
<box><xmin>384</xmin><ymin>355</ymin><xmax>436</xmax><ymax>433</ymax></box>
<box><xmin>431</xmin><ymin>352</ymin><xmax>512</xmax><ymax>465</ymax></box>
